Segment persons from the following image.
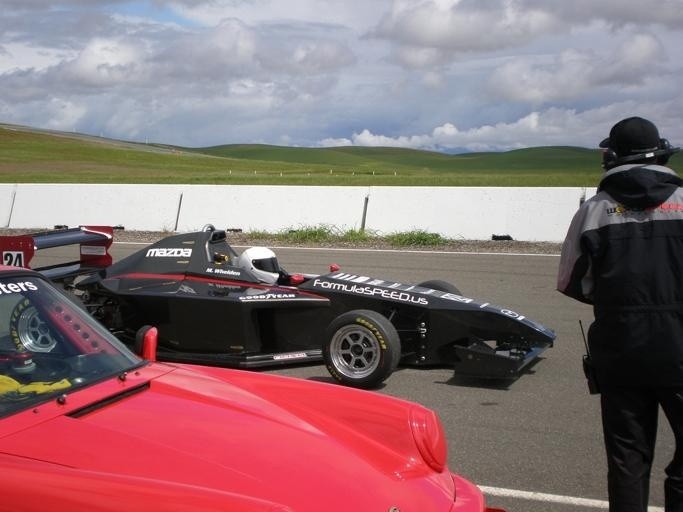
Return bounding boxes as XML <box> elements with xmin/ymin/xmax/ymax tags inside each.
<box><xmin>555</xmin><ymin>113</ymin><xmax>682</xmax><ymax>512</ymax></box>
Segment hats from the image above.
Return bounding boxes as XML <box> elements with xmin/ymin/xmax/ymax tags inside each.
<box><xmin>598</xmin><ymin>115</ymin><xmax>661</xmax><ymax>161</ymax></box>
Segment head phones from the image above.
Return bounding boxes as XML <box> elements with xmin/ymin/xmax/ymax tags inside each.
<box><xmin>601</xmin><ymin>138</ymin><xmax>680</xmax><ymax>172</ymax></box>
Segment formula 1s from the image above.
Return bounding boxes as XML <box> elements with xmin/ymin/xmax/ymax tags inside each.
<box><xmin>1</xmin><ymin>225</ymin><xmax>556</xmax><ymax>388</ymax></box>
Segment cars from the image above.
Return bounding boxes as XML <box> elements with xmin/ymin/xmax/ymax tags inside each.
<box><xmin>3</xmin><ymin>266</ymin><xmax>485</xmax><ymax>512</ymax></box>
